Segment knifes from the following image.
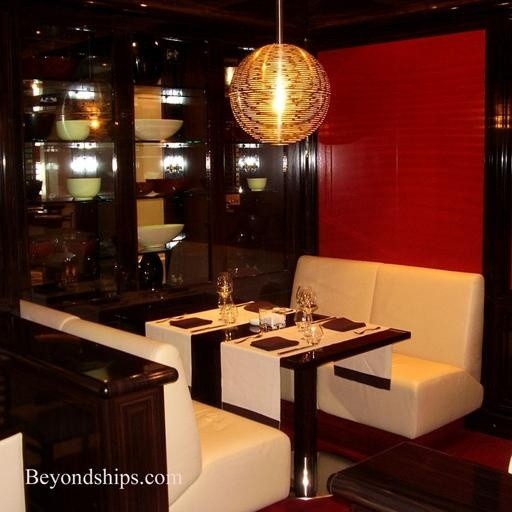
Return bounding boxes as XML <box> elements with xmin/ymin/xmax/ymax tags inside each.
<box><xmin>191</xmin><ymin>324</ymin><xmax>226</xmax><ymax>334</ymax></box>
<box><xmin>157</xmin><ymin>315</ymin><xmax>183</xmax><ymax>324</ymax></box>
<box><xmin>311</xmin><ymin>316</ymin><xmax>336</xmax><ymax>325</ymax></box>
<box><xmin>235</xmin><ymin>338</ymin><xmax>249</xmax><ymax>345</ymax></box>
<box><xmin>236</xmin><ymin>303</ymin><xmax>248</xmax><ymax>308</ymax></box>
<box><xmin>278</xmin><ymin>345</ymin><xmax>312</xmax><ymax>354</ymax></box>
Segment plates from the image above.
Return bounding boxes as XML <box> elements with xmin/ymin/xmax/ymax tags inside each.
<box><xmin>250</xmin><ymin>325</ymin><xmax>260</xmax><ymax>333</ymax></box>
<box><xmin>97</xmin><ymin>192</ymin><xmax>113</xmax><ymax>201</ymax></box>
<box><xmin>250</xmin><ymin>319</ymin><xmax>260</xmax><ymax>326</ymax></box>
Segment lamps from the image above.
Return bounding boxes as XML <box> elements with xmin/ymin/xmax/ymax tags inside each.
<box><xmin>228</xmin><ymin>0</ymin><xmax>332</xmax><ymax>146</ymax></box>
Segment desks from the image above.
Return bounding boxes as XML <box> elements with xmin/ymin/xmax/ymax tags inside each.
<box><xmin>0</xmin><ymin>312</ymin><xmax>177</xmax><ymax>511</ymax></box>
<box><xmin>145</xmin><ymin>299</ymin><xmax>410</xmax><ymax>496</ymax></box>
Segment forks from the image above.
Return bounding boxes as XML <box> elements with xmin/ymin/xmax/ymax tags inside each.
<box><xmin>354</xmin><ymin>325</ymin><xmax>381</xmax><ymax>335</ymax></box>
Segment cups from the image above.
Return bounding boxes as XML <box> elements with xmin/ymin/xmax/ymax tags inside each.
<box><xmin>169</xmin><ymin>270</ymin><xmax>186</xmax><ymax>290</ymax></box>
<box><xmin>257</xmin><ymin>307</ymin><xmax>286</xmax><ymax>333</ymax></box>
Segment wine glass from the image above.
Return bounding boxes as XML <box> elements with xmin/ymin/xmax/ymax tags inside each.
<box><xmin>293</xmin><ymin>285</ymin><xmax>325</xmax><ymax>357</ymax></box>
<box><xmin>217</xmin><ymin>274</ymin><xmax>237</xmax><ymax>343</ymax></box>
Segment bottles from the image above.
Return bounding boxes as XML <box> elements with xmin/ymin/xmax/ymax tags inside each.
<box><xmin>29</xmin><ymin>236</ymin><xmax>122</xmax><ymax>292</ymax></box>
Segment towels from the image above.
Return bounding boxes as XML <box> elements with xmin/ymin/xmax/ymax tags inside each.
<box><xmin>322</xmin><ymin>317</ymin><xmax>365</xmax><ymax>332</ymax></box>
<box><xmin>250</xmin><ymin>336</ymin><xmax>299</xmax><ymax>351</ymax></box>
<box><xmin>170</xmin><ymin>318</ymin><xmax>212</xmax><ymax>329</ymax></box>
<box><xmin>244</xmin><ymin>300</ymin><xmax>278</xmax><ymax>313</ymax></box>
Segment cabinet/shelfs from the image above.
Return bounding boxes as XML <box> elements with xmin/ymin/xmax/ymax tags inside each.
<box><xmin>2</xmin><ymin>0</ymin><xmax>306</xmax><ymax>330</ymax></box>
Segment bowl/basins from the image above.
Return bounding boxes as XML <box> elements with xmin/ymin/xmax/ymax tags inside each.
<box><xmin>56</xmin><ymin>119</ymin><xmax>89</xmax><ymax>141</ymax></box>
<box><xmin>138</xmin><ymin>223</ymin><xmax>184</xmax><ymax>248</ymax></box>
<box><xmin>23</xmin><ymin>111</ymin><xmax>57</xmax><ymax>139</ymax></box>
<box><xmin>65</xmin><ymin>177</ymin><xmax>102</xmax><ymax>201</ymax></box>
<box><xmin>136</xmin><ymin>181</ymin><xmax>154</xmax><ymax>196</ymax></box>
<box><xmin>24</xmin><ymin>178</ymin><xmax>42</xmax><ymax>201</ymax></box>
<box><xmin>134</xmin><ymin>117</ymin><xmax>184</xmax><ymax>141</ymax></box>
<box><xmin>246</xmin><ymin>177</ymin><xmax>268</xmax><ymax>192</ymax></box>
<box><xmin>146</xmin><ymin>176</ymin><xmax>191</xmax><ymax>195</ymax></box>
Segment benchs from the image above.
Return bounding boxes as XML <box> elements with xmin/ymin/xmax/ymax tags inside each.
<box><xmin>281</xmin><ymin>255</ymin><xmax>485</xmax><ymax>462</ymax></box>
<box><xmin>20</xmin><ymin>297</ymin><xmax>291</xmax><ymax>511</ymax></box>
